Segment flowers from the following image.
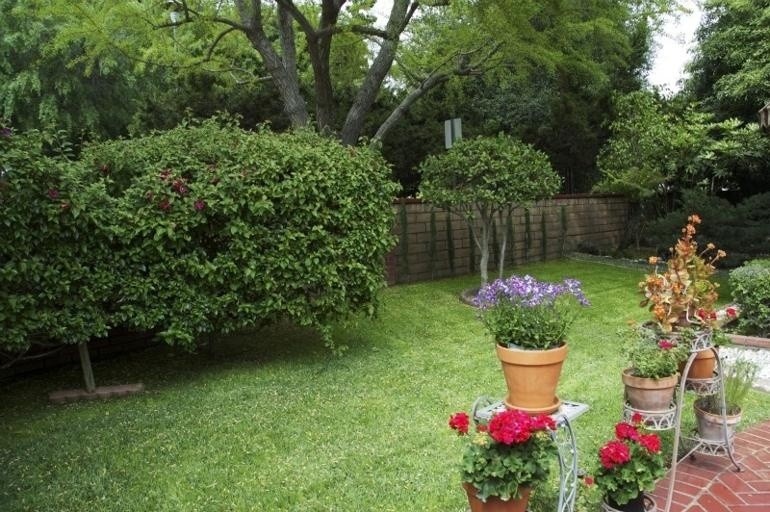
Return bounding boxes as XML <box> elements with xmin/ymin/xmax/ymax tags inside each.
<box><xmin>616</xmin><ymin>320</ymin><xmax>689</xmax><ymax>380</ymax></box>
<box><xmin>639</xmin><ymin>215</ymin><xmax>727</xmax><ymax>332</ymax></box>
<box><xmin>472</xmin><ymin>273</ymin><xmax>592</xmax><ymax>350</ymax></box>
<box><xmin>682</xmin><ymin>308</ymin><xmax>736</xmax><ymax>350</ymax></box>
<box><xmin>449</xmin><ymin>410</ymin><xmax>556</xmax><ymax>503</ymax></box>
<box><xmin>586</xmin><ymin>413</ymin><xmax>665</xmax><ymax>506</ymax></box>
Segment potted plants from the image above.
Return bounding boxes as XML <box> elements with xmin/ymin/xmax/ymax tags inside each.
<box><xmin>693</xmin><ymin>356</ymin><xmax>759</xmax><ymax>450</ymax></box>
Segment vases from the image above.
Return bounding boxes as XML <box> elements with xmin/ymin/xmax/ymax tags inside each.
<box><xmin>462</xmin><ymin>483</ymin><xmax>532</xmax><ymax>512</ymax></box>
<box><xmin>496</xmin><ymin>343</ymin><xmax>568</xmax><ymax>416</ymax></box>
<box><xmin>602</xmin><ymin>494</ymin><xmax>657</xmax><ymax>512</ymax></box>
<box><xmin>680</xmin><ymin>348</ymin><xmax>718</xmax><ymax>391</ymax></box>
<box><xmin>622</xmin><ymin>368</ymin><xmax>678</xmax><ymax>424</ymax></box>
<box><xmin>640</xmin><ymin>321</ymin><xmax>712</xmax><ymax>349</ymax></box>
